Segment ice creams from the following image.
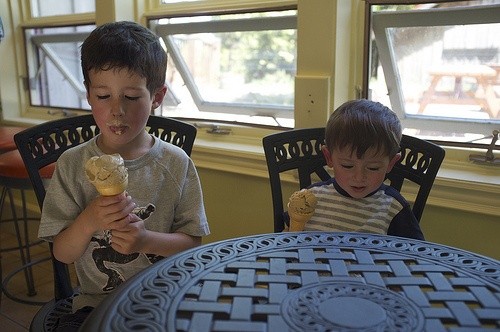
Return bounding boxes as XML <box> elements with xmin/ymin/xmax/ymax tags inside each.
<box><xmin>84</xmin><ymin>153</ymin><xmax>128</xmax><ymax>196</ymax></box>
<box><xmin>287</xmin><ymin>188</ymin><xmax>317</xmax><ymax>231</ymax></box>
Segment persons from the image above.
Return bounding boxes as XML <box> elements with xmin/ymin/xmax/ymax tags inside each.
<box><xmin>284</xmin><ymin>99</ymin><xmax>426</xmax><ymax>241</ymax></box>
<box><xmin>37</xmin><ymin>21</ymin><xmax>212</xmax><ymax>322</ymax></box>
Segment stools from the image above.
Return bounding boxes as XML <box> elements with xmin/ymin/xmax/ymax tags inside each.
<box><xmin>0</xmin><ymin>127</ymin><xmax>59</xmax><ymax>306</ymax></box>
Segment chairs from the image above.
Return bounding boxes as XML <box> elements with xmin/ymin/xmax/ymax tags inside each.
<box><xmin>262</xmin><ymin>127</ymin><xmax>445</xmax><ymax>232</ymax></box>
<box><xmin>14</xmin><ymin>114</ymin><xmax>197</xmax><ymax>332</ymax></box>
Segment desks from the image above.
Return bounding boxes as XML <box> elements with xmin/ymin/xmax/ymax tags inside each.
<box><xmin>78</xmin><ymin>230</ymin><xmax>500</xmax><ymax>332</ymax></box>
